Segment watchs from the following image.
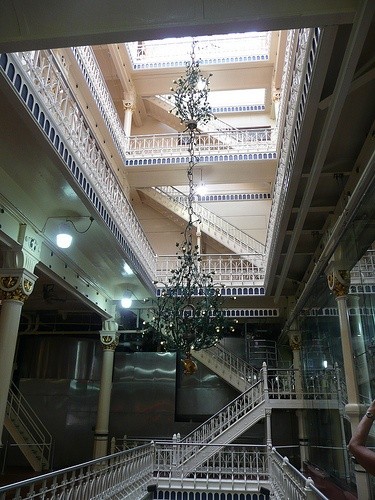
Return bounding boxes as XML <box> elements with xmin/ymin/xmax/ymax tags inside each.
<box><xmin>365</xmin><ymin>411</ymin><xmax>374</xmax><ymax>422</ymax></box>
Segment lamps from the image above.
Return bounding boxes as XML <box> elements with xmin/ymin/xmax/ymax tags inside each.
<box><xmin>140</xmin><ymin>37</ymin><xmax>237</xmax><ymax>352</ymax></box>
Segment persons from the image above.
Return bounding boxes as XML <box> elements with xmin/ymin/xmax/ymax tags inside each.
<box><xmin>348</xmin><ymin>400</ymin><xmax>375</xmax><ymax>478</ymax></box>
